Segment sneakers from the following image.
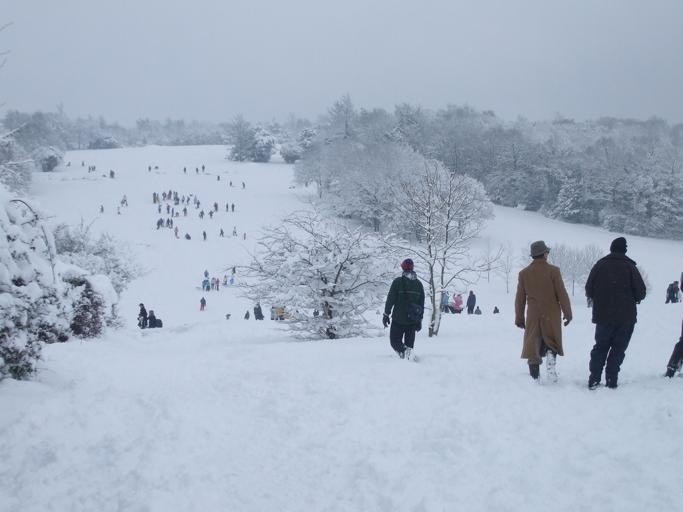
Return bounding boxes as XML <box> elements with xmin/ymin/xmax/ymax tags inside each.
<box><xmin>663</xmin><ymin>366</ymin><xmax>676</xmax><ymax>378</ymax></box>
<box><xmin>587</xmin><ymin>376</ymin><xmax>618</xmax><ymax>390</ymax></box>
<box><xmin>527</xmin><ymin>358</ymin><xmax>559</xmax><ymax>386</ymax></box>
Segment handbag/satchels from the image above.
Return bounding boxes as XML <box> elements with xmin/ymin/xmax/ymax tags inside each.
<box><xmin>405</xmin><ymin>302</ymin><xmax>423</xmax><ymax>322</ymax></box>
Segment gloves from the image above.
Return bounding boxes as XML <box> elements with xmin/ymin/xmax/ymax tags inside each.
<box><xmin>561</xmin><ymin>315</ymin><xmax>571</xmax><ymax>326</ymax></box>
<box><xmin>515</xmin><ymin>322</ymin><xmax>526</xmax><ymax>329</ymax></box>
<box><xmin>382</xmin><ymin>313</ymin><xmax>390</xmax><ymax>327</ymax></box>
<box><xmin>415</xmin><ymin>319</ymin><xmax>422</xmax><ymax>332</ymax></box>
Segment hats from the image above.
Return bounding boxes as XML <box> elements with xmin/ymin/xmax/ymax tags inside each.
<box><xmin>400</xmin><ymin>258</ymin><xmax>414</xmax><ymax>272</ymax></box>
<box><xmin>527</xmin><ymin>240</ymin><xmax>552</xmax><ymax>257</ymax></box>
<box><xmin>609</xmin><ymin>236</ymin><xmax>628</xmax><ymax>252</ymax></box>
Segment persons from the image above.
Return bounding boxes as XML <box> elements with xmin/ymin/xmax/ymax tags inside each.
<box><xmin>226</xmin><ymin>313</ymin><xmax>231</xmax><ymax>319</ymax></box>
<box><xmin>122</xmin><ymin>194</ymin><xmax>128</xmax><ymax>205</ymax></box>
<box><xmin>147</xmin><ymin>310</ymin><xmax>155</xmax><ymax>329</ymax></box>
<box><xmin>99</xmin><ymin>204</ymin><xmax>104</xmax><ymax>212</ymax></box>
<box><xmin>137</xmin><ymin>302</ymin><xmax>148</xmax><ymax>326</ymax></box>
<box><xmin>661</xmin><ymin>272</ymin><xmax>683</xmax><ymax>378</ymax></box>
<box><xmin>121</xmin><ymin>199</ymin><xmax>124</xmax><ymax>207</ymax></box>
<box><xmin>138</xmin><ymin>314</ymin><xmax>145</xmax><ymax>330</ymax></box>
<box><xmin>474</xmin><ymin>306</ymin><xmax>482</xmax><ymax>314</ymax></box>
<box><xmin>199</xmin><ymin>297</ymin><xmax>206</xmax><ymax>310</ymax></box>
<box><xmin>579</xmin><ymin>236</ymin><xmax>646</xmax><ymax>390</ymax></box>
<box><xmin>664</xmin><ymin>280</ymin><xmax>679</xmax><ymax>304</ymax></box>
<box><xmin>252</xmin><ymin>302</ymin><xmax>291</xmax><ymax>320</ymax></box>
<box><xmin>442</xmin><ymin>289</ymin><xmax>450</xmax><ymax>306</ymax></box>
<box><xmin>513</xmin><ymin>239</ymin><xmax>572</xmax><ymax>386</ymax></box>
<box><xmin>382</xmin><ymin>258</ymin><xmax>424</xmax><ymax>356</ymax></box>
<box><xmin>200</xmin><ymin>265</ymin><xmax>236</xmax><ymax>292</ymax></box>
<box><xmin>452</xmin><ymin>292</ymin><xmax>463</xmax><ymax>310</ymax></box>
<box><xmin>144</xmin><ymin>161</ymin><xmax>248</xmax><ymax>241</ymax></box>
<box><xmin>244</xmin><ymin>310</ymin><xmax>250</xmax><ymax>319</ymax></box>
<box><xmin>494</xmin><ymin>306</ymin><xmax>499</xmax><ymax>314</ymax></box>
<box><xmin>117</xmin><ymin>206</ymin><xmax>121</xmax><ymax>215</ymax></box>
<box><xmin>467</xmin><ymin>290</ymin><xmax>476</xmax><ymax>314</ymax></box>
